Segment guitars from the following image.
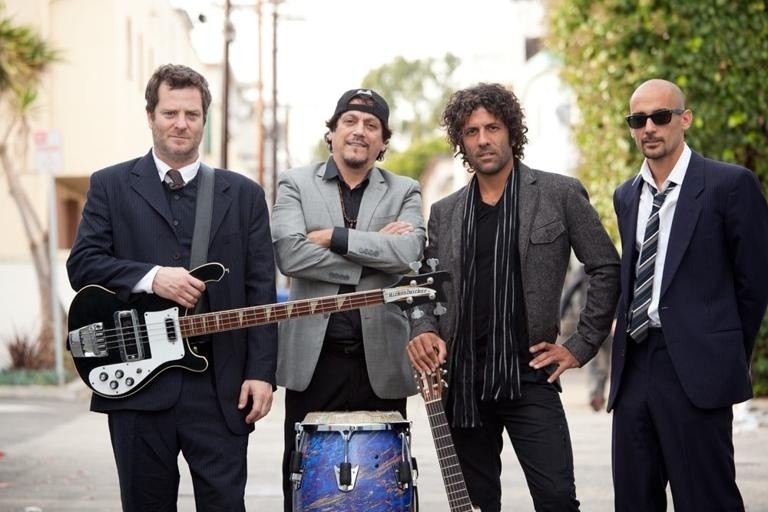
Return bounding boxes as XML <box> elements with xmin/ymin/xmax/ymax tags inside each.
<box><xmin>412</xmin><ymin>349</ymin><xmax>480</xmax><ymax>512</ymax></box>
<box><xmin>67</xmin><ymin>258</ymin><xmax>452</xmax><ymax>400</ymax></box>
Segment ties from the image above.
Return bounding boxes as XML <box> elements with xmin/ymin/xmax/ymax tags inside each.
<box><xmin>167</xmin><ymin>170</ymin><xmax>184</xmax><ymax>189</ymax></box>
<box><xmin>630</xmin><ymin>182</ymin><xmax>678</xmax><ymax>344</ymax></box>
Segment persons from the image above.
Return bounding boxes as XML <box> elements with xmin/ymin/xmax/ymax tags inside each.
<box><xmin>404</xmin><ymin>80</ymin><xmax>624</xmax><ymax>512</ymax></box>
<box><xmin>267</xmin><ymin>85</ymin><xmax>427</xmax><ymax>512</ymax></box>
<box><xmin>65</xmin><ymin>63</ymin><xmax>280</xmax><ymax>512</ymax></box>
<box><xmin>608</xmin><ymin>77</ymin><xmax>767</xmax><ymax>512</ymax></box>
<box><xmin>559</xmin><ymin>266</ymin><xmax>616</xmax><ymax>413</ymax></box>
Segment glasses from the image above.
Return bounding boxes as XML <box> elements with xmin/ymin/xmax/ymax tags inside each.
<box><xmin>626</xmin><ymin>110</ymin><xmax>684</xmax><ymax>128</ymax></box>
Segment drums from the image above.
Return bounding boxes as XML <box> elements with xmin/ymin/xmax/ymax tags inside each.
<box><xmin>289</xmin><ymin>410</ymin><xmax>419</xmax><ymax>512</ymax></box>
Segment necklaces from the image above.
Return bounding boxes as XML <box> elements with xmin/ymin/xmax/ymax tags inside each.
<box><xmin>337</xmin><ymin>180</ymin><xmax>359</xmax><ymax>228</ymax></box>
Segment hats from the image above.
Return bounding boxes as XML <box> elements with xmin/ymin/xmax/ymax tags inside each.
<box><xmin>335</xmin><ymin>90</ymin><xmax>389</xmax><ymax>129</ymax></box>
